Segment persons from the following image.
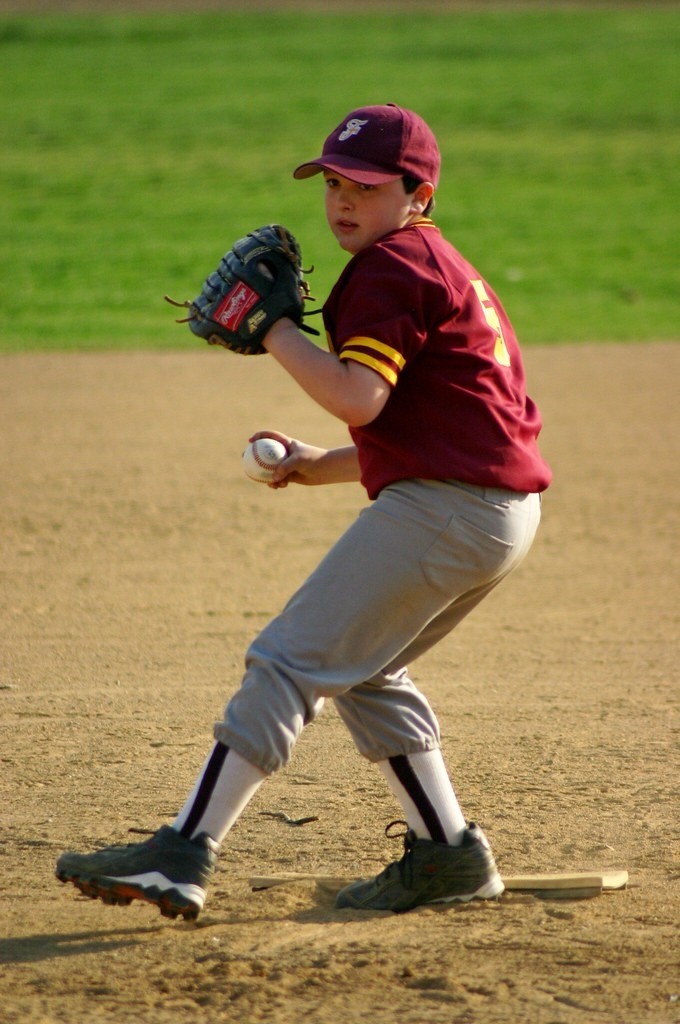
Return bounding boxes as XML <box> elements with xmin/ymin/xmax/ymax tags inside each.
<box><xmin>52</xmin><ymin>104</ymin><xmax>552</xmax><ymax>924</ymax></box>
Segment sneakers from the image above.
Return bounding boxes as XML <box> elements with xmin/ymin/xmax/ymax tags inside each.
<box><xmin>55</xmin><ymin>825</ymin><xmax>221</xmax><ymax>921</ymax></box>
<box><xmin>335</xmin><ymin>820</ymin><xmax>505</xmax><ymax>913</ymax></box>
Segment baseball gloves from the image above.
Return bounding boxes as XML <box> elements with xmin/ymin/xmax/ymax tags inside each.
<box><xmin>165</xmin><ymin>225</ymin><xmax>326</xmax><ymax>354</ymax></box>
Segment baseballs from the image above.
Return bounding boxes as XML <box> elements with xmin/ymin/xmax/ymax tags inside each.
<box><xmin>242</xmin><ymin>438</ymin><xmax>289</xmax><ymax>483</ymax></box>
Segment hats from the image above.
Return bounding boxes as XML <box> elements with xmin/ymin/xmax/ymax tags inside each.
<box><xmin>294</xmin><ymin>103</ymin><xmax>441</xmax><ymax>191</ymax></box>
